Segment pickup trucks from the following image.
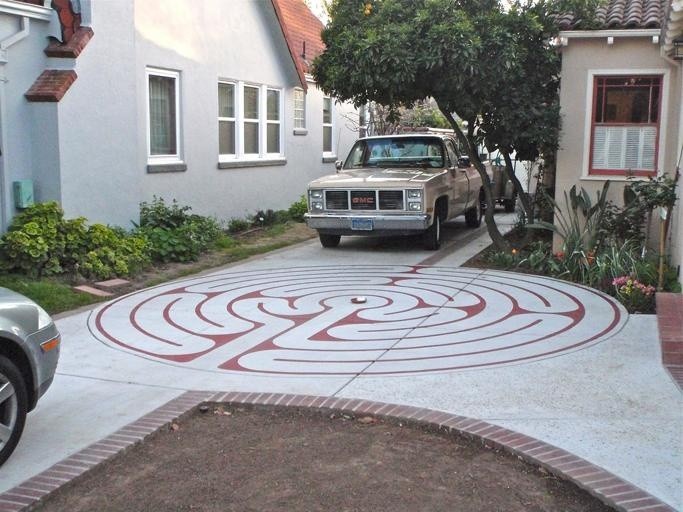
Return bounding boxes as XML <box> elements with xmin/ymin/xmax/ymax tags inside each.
<box><xmin>302</xmin><ymin>132</ymin><xmax>495</xmax><ymax>251</ymax></box>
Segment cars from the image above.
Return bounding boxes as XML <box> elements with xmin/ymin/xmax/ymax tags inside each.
<box><xmin>0</xmin><ymin>281</ymin><xmax>63</xmax><ymax>466</ymax></box>
